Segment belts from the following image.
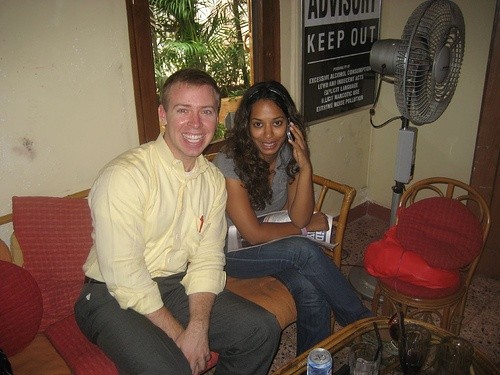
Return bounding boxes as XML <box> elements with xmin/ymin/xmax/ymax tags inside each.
<box><xmin>83</xmin><ymin>276</ymin><xmax>104</xmax><ymax>284</ymax></box>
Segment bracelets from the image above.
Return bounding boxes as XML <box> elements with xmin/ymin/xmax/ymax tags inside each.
<box><xmin>301</xmin><ymin>227</ymin><xmax>308</xmax><ymax>236</ymax></box>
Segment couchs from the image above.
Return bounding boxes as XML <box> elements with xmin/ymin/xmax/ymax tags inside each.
<box><xmin>1</xmin><ymin>153</ymin><xmax>356</xmax><ymax>375</ymax></box>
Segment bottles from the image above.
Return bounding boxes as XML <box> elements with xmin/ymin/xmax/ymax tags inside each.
<box><xmin>307</xmin><ymin>347</ymin><xmax>333</xmax><ymax>375</ymax></box>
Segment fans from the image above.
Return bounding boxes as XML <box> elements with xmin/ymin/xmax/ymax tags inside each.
<box><xmin>346</xmin><ymin>0</ymin><xmax>467</xmax><ymax>305</ymax></box>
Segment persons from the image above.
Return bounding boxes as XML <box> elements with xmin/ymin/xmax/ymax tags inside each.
<box><xmin>72</xmin><ymin>68</ymin><xmax>284</xmax><ymax>375</ymax></box>
<box><xmin>210</xmin><ymin>80</ymin><xmax>377</xmax><ymax>354</ymax></box>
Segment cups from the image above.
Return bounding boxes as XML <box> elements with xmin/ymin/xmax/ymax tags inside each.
<box><xmin>436</xmin><ymin>335</ymin><xmax>476</xmax><ymax>374</ymax></box>
<box><xmin>346</xmin><ymin>340</ymin><xmax>383</xmax><ymax>374</ymax></box>
<box><xmin>396</xmin><ymin>321</ymin><xmax>432</xmax><ymax>375</ymax></box>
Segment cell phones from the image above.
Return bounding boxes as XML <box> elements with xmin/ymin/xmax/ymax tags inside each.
<box><xmin>286</xmin><ymin>116</ymin><xmax>296</xmax><ymax>142</ymax></box>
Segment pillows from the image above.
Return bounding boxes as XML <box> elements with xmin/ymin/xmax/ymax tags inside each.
<box><xmin>0</xmin><ymin>261</ymin><xmax>44</xmax><ymax>358</ymax></box>
<box><xmin>12</xmin><ymin>196</ymin><xmax>95</xmax><ymax>332</ymax></box>
<box><xmin>44</xmin><ymin>313</ymin><xmax>220</xmax><ymax>375</ymax></box>
<box><xmin>396</xmin><ymin>197</ymin><xmax>484</xmax><ymax>272</ymax></box>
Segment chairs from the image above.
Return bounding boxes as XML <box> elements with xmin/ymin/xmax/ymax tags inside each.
<box><xmin>370</xmin><ymin>176</ymin><xmax>492</xmax><ymax>336</ymax></box>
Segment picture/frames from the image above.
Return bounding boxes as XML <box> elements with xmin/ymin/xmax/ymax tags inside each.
<box><xmin>299</xmin><ymin>0</ymin><xmax>383</xmax><ymax>129</ymax></box>
